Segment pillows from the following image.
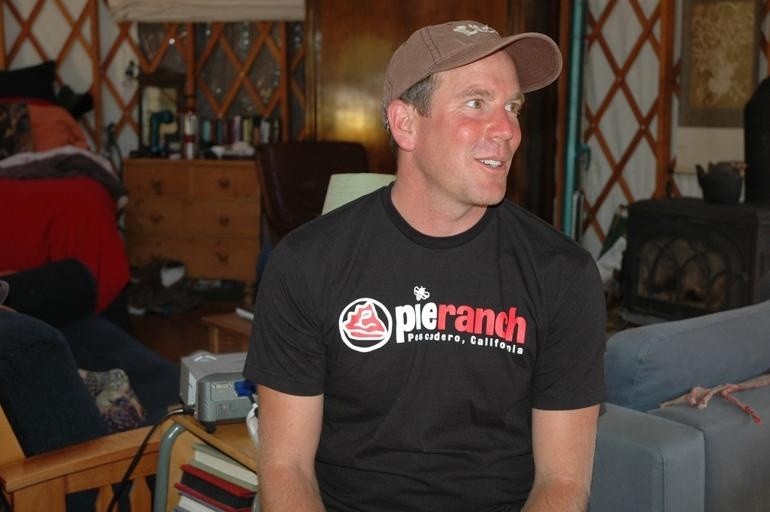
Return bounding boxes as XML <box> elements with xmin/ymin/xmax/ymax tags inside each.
<box><xmin>1</xmin><ymin>59</ymin><xmax>94</xmax><ymax>160</ymax></box>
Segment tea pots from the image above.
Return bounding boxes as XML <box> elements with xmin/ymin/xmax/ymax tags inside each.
<box><xmin>693</xmin><ymin>159</ymin><xmax>746</xmax><ymax>203</ymax></box>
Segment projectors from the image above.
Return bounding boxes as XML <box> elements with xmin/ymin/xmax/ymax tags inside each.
<box><xmin>176</xmin><ymin>350</ymin><xmax>258</xmax><ymax>427</ymax></box>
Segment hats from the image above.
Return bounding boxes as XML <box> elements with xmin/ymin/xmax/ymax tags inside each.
<box><xmin>381</xmin><ymin>21</ymin><xmax>563</xmax><ymax>106</ymax></box>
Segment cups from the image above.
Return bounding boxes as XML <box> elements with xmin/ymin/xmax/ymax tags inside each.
<box><xmin>164</xmin><ymin>133</ymin><xmax>183</xmax><ymax>161</ymax></box>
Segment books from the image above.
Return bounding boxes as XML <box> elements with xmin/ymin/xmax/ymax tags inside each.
<box><xmin>173</xmin><ymin>442</ymin><xmax>259</xmax><ymax>512</ymax></box>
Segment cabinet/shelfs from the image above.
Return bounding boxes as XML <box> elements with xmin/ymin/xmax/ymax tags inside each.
<box><xmin>615</xmin><ymin>195</ymin><xmax>770</xmax><ymax>331</ymax></box>
<box><xmin>121</xmin><ymin>155</ymin><xmax>264</xmax><ymax>308</ymax></box>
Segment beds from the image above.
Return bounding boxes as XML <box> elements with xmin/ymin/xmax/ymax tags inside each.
<box><xmin>0</xmin><ymin>152</ymin><xmax>132</xmax><ymax>341</ymax></box>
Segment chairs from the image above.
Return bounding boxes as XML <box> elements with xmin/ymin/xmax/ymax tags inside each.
<box><xmin>0</xmin><ymin>306</ymin><xmax>168</xmax><ymax>512</ymax></box>
<box><xmin>254</xmin><ymin>141</ymin><xmax>369</xmax><ymax>304</ymax></box>
<box><xmin>586</xmin><ymin>299</ymin><xmax>770</xmax><ymax>512</ymax></box>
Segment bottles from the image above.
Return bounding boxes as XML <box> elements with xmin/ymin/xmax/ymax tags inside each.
<box><xmin>178</xmin><ymin>107</ymin><xmax>281</xmax><ymax>161</ymax></box>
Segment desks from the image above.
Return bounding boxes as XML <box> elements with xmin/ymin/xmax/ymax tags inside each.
<box><xmin>152</xmin><ymin>403</ymin><xmax>262</xmax><ymax>512</ymax></box>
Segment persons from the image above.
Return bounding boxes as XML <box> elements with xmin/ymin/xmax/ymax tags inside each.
<box><xmin>242</xmin><ymin>19</ymin><xmax>608</xmax><ymax>512</ymax></box>
